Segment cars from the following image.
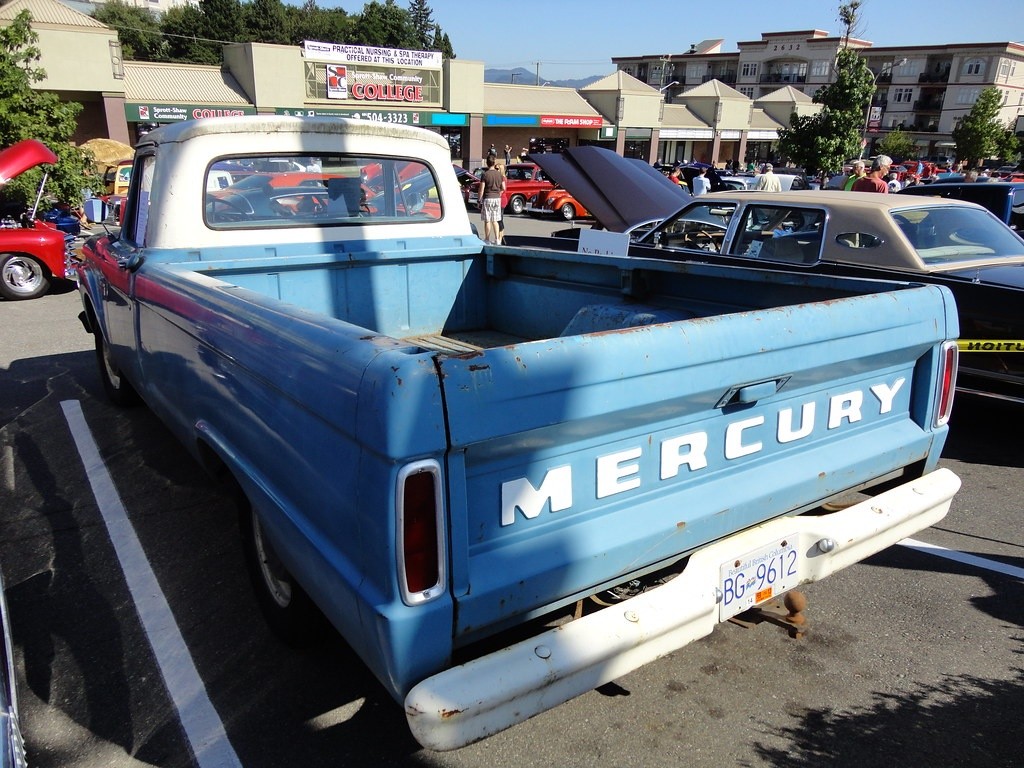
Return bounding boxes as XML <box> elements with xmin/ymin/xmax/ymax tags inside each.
<box><xmin>0</xmin><ymin>135</ymin><xmax>68</xmax><ymax>303</ymax></box>
<box><xmin>208</xmin><ymin>125</ymin><xmax>1024</xmax><ymax>245</ymax></box>
<box><xmin>517</xmin><ymin>144</ymin><xmax>1024</xmax><ymax>417</ymax></box>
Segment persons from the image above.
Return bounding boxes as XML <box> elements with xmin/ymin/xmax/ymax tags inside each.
<box><xmin>495</xmin><ymin>164</ymin><xmax>507</xmax><ymax>243</ymax></box>
<box><xmin>488</xmin><ymin>141</ymin><xmax>529</xmax><ymax>165</ymax></box>
<box><xmin>304</xmin><ymin>157</ymin><xmax>323</xmax><ymax>189</ymax></box>
<box><xmin>476</xmin><ymin>154</ymin><xmax>503</xmax><ymax>246</ymax></box>
<box><xmin>653</xmin><ymin>155</ymin><xmax>1012</xmax><ymax>196</ymax></box>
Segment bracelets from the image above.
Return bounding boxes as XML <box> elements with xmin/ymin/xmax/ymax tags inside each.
<box><xmin>477</xmin><ymin>200</ymin><xmax>481</xmax><ymax>203</ymax></box>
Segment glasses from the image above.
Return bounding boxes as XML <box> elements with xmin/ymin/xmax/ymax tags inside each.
<box><xmin>495</xmin><ymin>168</ymin><xmax>499</xmax><ymax>171</ymax></box>
<box><xmin>853</xmin><ymin>167</ymin><xmax>862</xmax><ymax>169</ymax></box>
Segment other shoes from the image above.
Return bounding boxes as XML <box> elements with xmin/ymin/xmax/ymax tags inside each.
<box><xmin>483</xmin><ymin>240</ymin><xmax>491</xmax><ymax>245</ymax></box>
<box><xmin>495</xmin><ymin>239</ymin><xmax>500</xmax><ymax>245</ymax></box>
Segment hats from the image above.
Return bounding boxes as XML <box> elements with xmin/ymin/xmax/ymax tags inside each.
<box><xmin>913</xmin><ymin>175</ymin><xmax>921</xmax><ymax>179</ymax></box>
<box><xmin>764</xmin><ymin>163</ymin><xmax>773</xmax><ymax>170</ymax></box>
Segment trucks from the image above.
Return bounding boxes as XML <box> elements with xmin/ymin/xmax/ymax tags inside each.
<box><xmin>72</xmin><ymin>113</ymin><xmax>965</xmax><ymax>755</ymax></box>
